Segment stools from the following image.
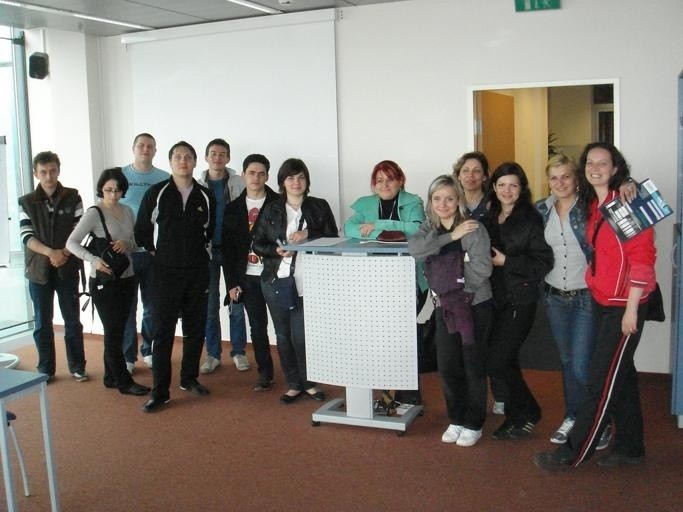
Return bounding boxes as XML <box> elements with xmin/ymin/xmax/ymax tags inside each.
<box><xmin>3</xmin><ymin>409</ymin><xmax>31</xmax><ymax>496</ymax></box>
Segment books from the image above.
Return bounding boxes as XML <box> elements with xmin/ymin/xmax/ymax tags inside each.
<box><xmin>599</xmin><ymin>177</ymin><xmax>674</xmax><ymax>244</ymax></box>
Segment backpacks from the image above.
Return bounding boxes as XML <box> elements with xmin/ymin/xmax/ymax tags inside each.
<box><xmin>72</xmin><ymin>206</ymin><xmax>130</xmax><ymax>298</ymax></box>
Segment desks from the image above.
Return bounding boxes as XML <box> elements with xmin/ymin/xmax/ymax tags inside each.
<box><xmin>0</xmin><ymin>365</ymin><xmax>61</xmax><ymax>512</ymax></box>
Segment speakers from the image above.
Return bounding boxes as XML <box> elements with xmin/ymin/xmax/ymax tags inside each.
<box><xmin>29</xmin><ymin>52</ymin><xmax>48</xmax><ymax>79</ymax></box>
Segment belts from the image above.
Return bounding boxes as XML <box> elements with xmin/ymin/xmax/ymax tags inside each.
<box><xmin>544</xmin><ymin>283</ymin><xmax>589</xmax><ymax>297</ymax></box>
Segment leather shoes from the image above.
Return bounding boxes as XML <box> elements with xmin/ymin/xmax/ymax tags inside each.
<box><xmin>304</xmin><ymin>388</ymin><xmax>325</xmax><ymax>401</ymax></box>
<box><xmin>280</xmin><ymin>390</ymin><xmax>303</xmax><ymax>404</ymax></box>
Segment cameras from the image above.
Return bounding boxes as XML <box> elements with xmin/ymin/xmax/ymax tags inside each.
<box><xmin>236</xmin><ymin>291</ymin><xmax>241</xmax><ymax>301</ymax></box>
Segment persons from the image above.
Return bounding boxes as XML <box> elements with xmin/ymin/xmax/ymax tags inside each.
<box><xmin>409</xmin><ymin>175</ymin><xmax>495</xmax><ymax>447</ymax></box>
<box><xmin>535</xmin><ymin>153</ymin><xmax>637</xmax><ymax>450</ymax></box>
<box><xmin>343</xmin><ymin>160</ymin><xmax>437</xmax><ymax>413</ymax></box>
<box><xmin>453</xmin><ymin>151</ymin><xmax>510</xmax><ymax>414</ymax></box>
<box><xmin>479</xmin><ymin>162</ymin><xmax>555</xmax><ymax>440</ymax></box>
<box><xmin>533</xmin><ymin>143</ymin><xmax>657</xmax><ymax>472</ymax></box>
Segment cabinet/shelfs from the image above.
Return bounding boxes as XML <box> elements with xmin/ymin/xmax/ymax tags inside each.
<box><xmin>669</xmin><ymin>68</ymin><xmax>682</xmax><ymax>428</ymax></box>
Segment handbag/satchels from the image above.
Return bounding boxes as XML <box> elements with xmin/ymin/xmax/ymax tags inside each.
<box><xmin>272</xmin><ymin>276</ymin><xmax>299</xmax><ymax>312</ymax></box>
<box><xmin>645</xmin><ymin>282</ymin><xmax>666</xmax><ymax>321</ymax></box>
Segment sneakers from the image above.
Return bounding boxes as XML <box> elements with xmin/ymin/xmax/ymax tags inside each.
<box><xmin>75</xmin><ymin>368</ymin><xmax>88</xmax><ymax>382</ymax></box>
<box><xmin>142</xmin><ymin>400</ymin><xmax>170</xmax><ymax>412</ymax></box>
<box><xmin>255</xmin><ymin>375</ymin><xmax>273</xmax><ymax>391</ymax></box>
<box><xmin>180</xmin><ymin>379</ymin><xmax>210</xmax><ymax>395</ymax></box>
<box><xmin>534</xmin><ymin>418</ymin><xmax>643</xmax><ymax>473</ymax></box>
<box><xmin>234</xmin><ymin>354</ymin><xmax>249</xmax><ymax>372</ymax></box>
<box><xmin>104</xmin><ymin>355</ymin><xmax>153</xmax><ymax>395</ymax></box>
<box><xmin>441</xmin><ymin>402</ymin><xmax>541</xmax><ymax>446</ymax></box>
<box><xmin>200</xmin><ymin>356</ymin><xmax>220</xmax><ymax>373</ymax></box>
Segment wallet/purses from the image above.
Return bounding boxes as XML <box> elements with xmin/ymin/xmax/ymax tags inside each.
<box><xmin>377</xmin><ymin>230</ymin><xmax>407</xmax><ymax>242</ymax></box>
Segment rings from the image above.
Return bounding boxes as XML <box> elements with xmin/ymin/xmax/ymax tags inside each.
<box><xmin>629</xmin><ymin>329</ymin><xmax>632</xmax><ymax>331</ymax></box>
